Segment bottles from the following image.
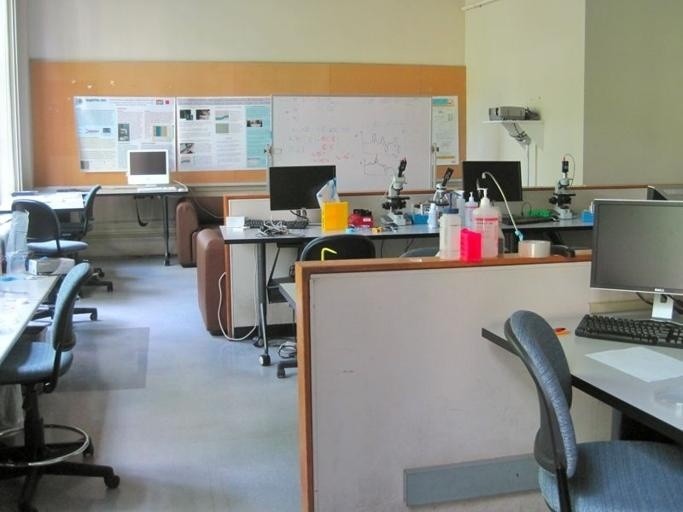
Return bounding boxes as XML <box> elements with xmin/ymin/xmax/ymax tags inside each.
<box><xmin>428</xmin><ymin>202</ymin><xmax>440</xmax><ymax>229</ymax></box>
<box><xmin>438</xmin><ymin>209</ymin><xmax>460</xmax><ymax>261</ymax></box>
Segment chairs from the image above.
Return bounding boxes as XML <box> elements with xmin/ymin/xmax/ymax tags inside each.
<box><xmin>59</xmin><ymin>184</ymin><xmax>112</xmax><ymax>299</ymax></box>
<box><xmin>11</xmin><ymin>198</ymin><xmax>97</xmax><ymax>321</ymax></box>
<box><xmin>504</xmin><ymin>307</ymin><xmax>683</xmax><ymax>511</ymax></box>
<box><xmin>0</xmin><ymin>263</ymin><xmax>120</xmax><ymax>511</ymax></box>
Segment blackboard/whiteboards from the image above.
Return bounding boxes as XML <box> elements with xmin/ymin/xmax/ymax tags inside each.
<box><xmin>272</xmin><ymin>95</ymin><xmax>432</xmax><ymax>196</ymax></box>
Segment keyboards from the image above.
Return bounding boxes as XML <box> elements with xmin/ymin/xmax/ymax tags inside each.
<box><xmin>575</xmin><ymin>314</ymin><xmax>683</xmax><ymax>349</ymax></box>
<box><xmin>502</xmin><ymin>216</ymin><xmax>552</xmax><ymax>224</ymax></box>
<box><xmin>250</xmin><ymin>220</ymin><xmax>307</xmax><ymax>229</ymax></box>
<box><xmin>137</xmin><ymin>187</ymin><xmax>176</xmax><ymax>192</ymax></box>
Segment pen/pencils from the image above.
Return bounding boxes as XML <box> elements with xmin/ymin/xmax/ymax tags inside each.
<box><xmin>552</xmin><ymin>328</ymin><xmax>566</xmax><ymax>333</ymax></box>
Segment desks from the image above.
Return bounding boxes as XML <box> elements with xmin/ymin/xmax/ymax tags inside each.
<box><xmin>481</xmin><ymin>300</ymin><xmax>683</xmax><ymax>440</ymax></box>
<box><xmin>0</xmin><ymin>189</ymin><xmax>85</xmax><ymax>214</ymax></box>
<box><xmin>34</xmin><ymin>185</ymin><xmax>188</xmax><ymax>266</ymax></box>
<box><xmin>0</xmin><ymin>274</ymin><xmax>59</xmax><ymax>371</ymax></box>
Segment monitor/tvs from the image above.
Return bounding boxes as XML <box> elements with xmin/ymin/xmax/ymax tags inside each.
<box><xmin>269</xmin><ymin>165</ymin><xmax>336</xmax><ymax>223</ymax></box>
<box><xmin>462</xmin><ymin>161</ymin><xmax>523</xmax><ymax>203</ymax></box>
<box><xmin>127</xmin><ymin>149</ymin><xmax>170</xmax><ymax>187</ymax></box>
<box><xmin>589</xmin><ymin>199</ymin><xmax>683</xmax><ymax>322</ymax></box>
<box><xmin>647</xmin><ymin>184</ymin><xmax>669</xmax><ymax>200</ymax></box>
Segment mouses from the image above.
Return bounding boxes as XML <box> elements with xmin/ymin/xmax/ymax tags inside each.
<box><xmin>178</xmin><ymin>188</ymin><xmax>184</xmax><ymax>192</ymax></box>
<box><xmin>549</xmin><ymin>215</ymin><xmax>559</xmax><ymax>222</ymax></box>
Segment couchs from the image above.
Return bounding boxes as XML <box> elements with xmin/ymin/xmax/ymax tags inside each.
<box><xmin>175</xmin><ymin>196</ymin><xmax>224</xmax><ymax>267</ymax></box>
<box><xmin>195</xmin><ymin>227</ymin><xmax>227</xmax><ymax>336</ymax></box>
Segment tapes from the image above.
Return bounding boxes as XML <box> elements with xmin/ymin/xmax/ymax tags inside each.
<box><xmin>518</xmin><ymin>240</ymin><xmax>551</xmax><ymax>258</ymax></box>
